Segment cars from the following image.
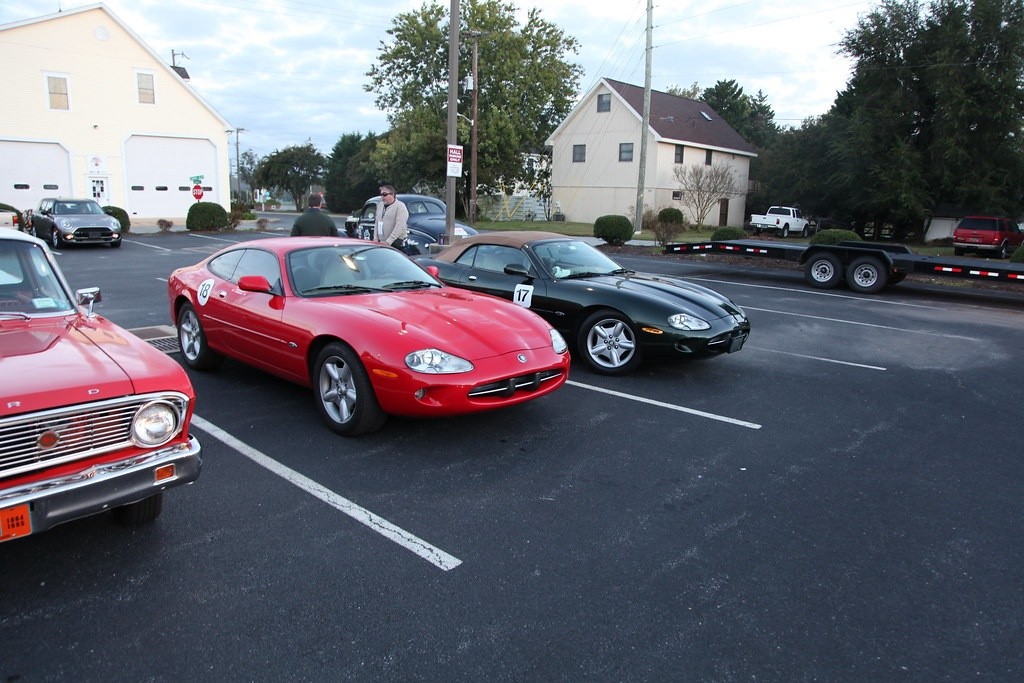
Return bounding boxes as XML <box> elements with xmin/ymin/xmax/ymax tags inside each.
<box><xmin>339</xmin><ymin>194</ymin><xmax>480</xmax><ymax>258</ymax></box>
<box><xmin>0</xmin><ymin>230</ymin><xmax>203</xmax><ymax>544</ymax></box>
<box><xmin>32</xmin><ymin>197</ymin><xmax>124</xmax><ymax>248</ymax></box>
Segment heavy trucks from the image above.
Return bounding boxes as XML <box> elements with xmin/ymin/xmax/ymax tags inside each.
<box><xmin>661</xmin><ymin>240</ymin><xmax>1024</xmax><ymax>295</ymax></box>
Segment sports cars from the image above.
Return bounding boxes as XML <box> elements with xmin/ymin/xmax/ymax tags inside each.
<box><xmin>416</xmin><ymin>230</ymin><xmax>752</xmax><ymax>378</ymax></box>
<box><xmin>165</xmin><ymin>235</ymin><xmax>572</xmax><ymax>437</ymax></box>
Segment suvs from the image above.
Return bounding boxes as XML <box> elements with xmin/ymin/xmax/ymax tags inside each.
<box><xmin>952</xmin><ymin>216</ymin><xmax>1024</xmax><ymax>258</ymax></box>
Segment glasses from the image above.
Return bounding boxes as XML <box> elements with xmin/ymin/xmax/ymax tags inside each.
<box><xmin>380</xmin><ymin>192</ymin><xmax>393</xmax><ymax>197</ymax></box>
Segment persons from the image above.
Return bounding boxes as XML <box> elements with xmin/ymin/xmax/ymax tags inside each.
<box><xmin>373</xmin><ymin>185</ymin><xmax>408</xmax><ymax>254</ymax></box>
<box><xmin>291</xmin><ymin>194</ymin><xmax>338</xmax><ymax>237</ymax></box>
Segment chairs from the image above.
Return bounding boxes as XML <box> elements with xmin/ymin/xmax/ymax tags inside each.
<box><xmin>292</xmin><ymin>265</ymin><xmax>321</xmax><ymax>291</ymax></box>
<box><xmin>331</xmin><ymin>254</ymin><xmax>373</xmax><ymax>285</ymax></box>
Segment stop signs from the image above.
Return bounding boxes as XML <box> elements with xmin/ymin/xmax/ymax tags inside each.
<box><xmin>192</xmin><ymin>186</ymin><xmax>203</xmax><ymax>199</ymax></box>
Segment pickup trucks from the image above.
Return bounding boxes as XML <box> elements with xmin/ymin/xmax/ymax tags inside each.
<box><xmin>750</xmin><ymin>206</ymin><xmax>810</xmax><ymax>238</ymax></box>
<box><xmin>0</xmin><ymin>210</ymin><xmax>21</xmax><ymax>231</ymax></box>
